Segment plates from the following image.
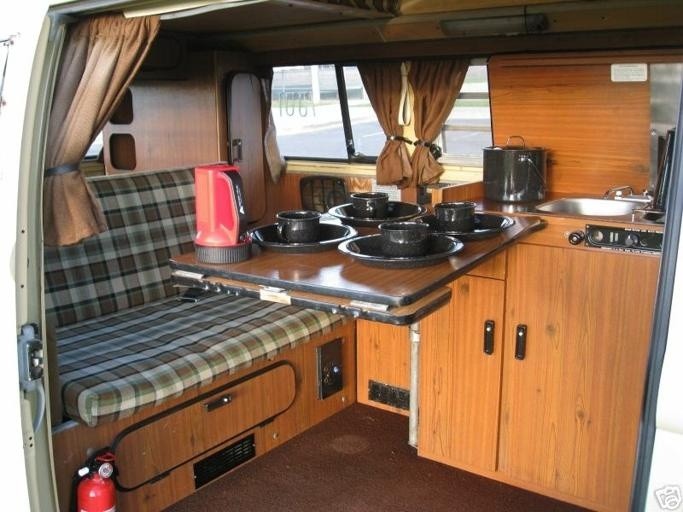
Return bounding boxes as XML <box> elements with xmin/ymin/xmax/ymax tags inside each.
<box><xmin>244</xmin><ymin>222</ymin><xmax>358</xmax><ymax>254</ymax></box>
<box><xmin>337</xmin><ymin>232</ymin><xmax>465</xmax><ymax>268</ymax></box>
<box><xmin>414</xmin><ymin>211</ymin><xmax>516</xmax><ymax>241</ymax></box>
<box><xmin>328</xmin><ymin>199</ymin><xmax>428</xmax><ymax>227</ymax></box>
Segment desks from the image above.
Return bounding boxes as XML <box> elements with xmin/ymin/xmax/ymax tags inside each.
<box><xmin>170</xmin><ymin>190</ymin><xmax>544</xmax><ymax>325</ymax></box>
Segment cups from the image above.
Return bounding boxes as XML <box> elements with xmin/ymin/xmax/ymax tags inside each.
<box><xmin>274</xmin><ymin>208</ymin><xmax>322</xmax><ymax>243</ymax></box>
<box><xmin>378</xmin><ymin>220</ymin><xmax>432</xmax><ymax>257</ymax></box>
<box><xmin>351</xmin><ymin>191</ymin><xmax>390</xmax><ymax>220</ymax></box>
<box><xmin>433</xmin><ymin>200</ymin><xmax>476</xmax><ymax>234</ymax></box>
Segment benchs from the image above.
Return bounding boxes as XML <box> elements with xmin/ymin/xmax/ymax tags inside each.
<box><xmin>38</xmin><ymin>162</ymin><xmax>356</xmax><ymax>511</ymax></box>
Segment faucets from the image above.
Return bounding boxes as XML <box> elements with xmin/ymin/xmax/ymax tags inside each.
<box><xmin>602</xmin><ymin>184</ymin><xmax>634</xmax><ymax>201</ymax></box>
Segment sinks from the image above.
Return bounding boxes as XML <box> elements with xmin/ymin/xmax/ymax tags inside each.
<box><xmin>533</xmin><ymin>196</ymin><xmax>651</xmax><ymax>220</ymax></box>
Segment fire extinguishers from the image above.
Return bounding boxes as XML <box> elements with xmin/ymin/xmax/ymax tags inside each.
<box><xmin>70</xmin><ymin>446</ymin><xmax>119</xmax><ymax>512</ymax></box>
